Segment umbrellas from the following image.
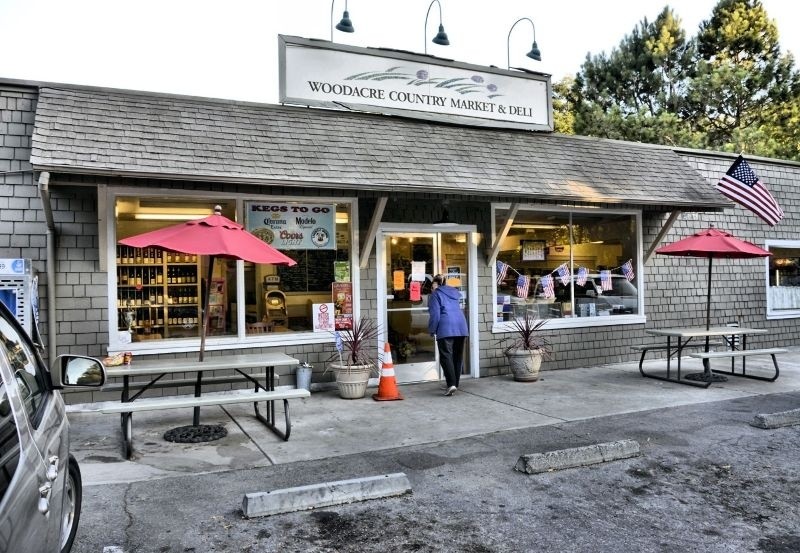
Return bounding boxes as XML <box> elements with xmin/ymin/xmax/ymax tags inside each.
<box><xmin>117</xmin><ymin>204</ymin><xmax>298</xmax><ymax>443</ymax></box>
<box><xmin>656</xmin><ymin>223</ymin><xmax>773</xmax><ymax>382</ymax></box>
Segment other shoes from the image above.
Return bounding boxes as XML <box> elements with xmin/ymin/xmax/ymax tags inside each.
<box><xmin>442</xmin><ymin>386</ymin><xmax>459</xmax><ymax>390</ymax></box>
<box><xmin>444</xmin><ymin>385</ymin><xmax>456</xmax><ymax>396</ymax></box>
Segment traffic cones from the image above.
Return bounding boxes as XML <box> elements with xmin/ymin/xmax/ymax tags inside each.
<box><xmin>371</xmin><ymin>342</ymin><xmax>404</xmax><ymax>401</ymax></box>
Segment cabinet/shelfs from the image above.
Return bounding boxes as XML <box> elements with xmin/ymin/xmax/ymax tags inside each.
<box><xmin>116</xmin><ymin>242</ymin><xmax>203</xmax><ymax>340</ymax></box>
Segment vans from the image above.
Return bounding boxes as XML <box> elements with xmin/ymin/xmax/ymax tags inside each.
<box><xmin>408</xmin><ymin>271</ymin><xmax>470</xmax><ymax>330</ymax></box>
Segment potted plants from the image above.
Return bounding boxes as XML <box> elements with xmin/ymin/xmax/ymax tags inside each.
<box><xmin>492</xmin><ymin>307</ymin><xmax>557</xmax><ymax>382</ymax></box>
<box><xmin>323</xmin><ymin>315</ymin><xmax>386</xmax><ymax>399</ymax></box>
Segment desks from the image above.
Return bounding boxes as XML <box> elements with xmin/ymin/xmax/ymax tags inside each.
<box><xmin>645</xmin><ymin>327</ymin><xmax>768</xmax><ymax>382</ymax></box>
<box><xmin>107</xmin><ymin>352</ymin><xmax>300</xmax><ymax>460</ymax></box>
<box><xmin>510</xmin><ymin>299</ymin><xmax>563</xmax><ymax>321</ymax></box>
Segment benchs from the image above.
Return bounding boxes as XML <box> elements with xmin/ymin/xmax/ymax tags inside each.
<box><xmin>101</xmin><ymin>372</ymin><xmax>310</xmax><ymax>414</ymax></box>
<box><xmin>629</xmin><ymin>339</ymin><xmax>789</xmax><ymax>358</ymax></box>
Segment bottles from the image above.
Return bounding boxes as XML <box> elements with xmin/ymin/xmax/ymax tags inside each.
<box><xmin>117</xmin><ymin>246</ymin><xmax>198</xmax><ymax>326</ymax></box>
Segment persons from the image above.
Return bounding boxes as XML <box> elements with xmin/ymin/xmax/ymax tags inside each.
<box><xmin>428</xmin><ymin>274</ymin><xmax>469</xmax><ymax>395</ymax></box>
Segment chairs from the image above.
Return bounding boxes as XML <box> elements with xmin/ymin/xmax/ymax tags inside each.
<box><xmin>247</xmin><ymin>321</ymin><xmax>275</xmax><ymax>334</ymax></box>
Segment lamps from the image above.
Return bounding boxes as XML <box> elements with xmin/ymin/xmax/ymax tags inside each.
<box><xmin>330</xmin><ymin>0</ymin><xmax>355</xmax><ymax>45</ymax></box>
<box><xmin>424</xmin><ymin>0</ymin><xmax>450</xmax><ymax>56</ymax></box>
<box><xmin>507</xmin><ymin>18</ymin><xmax>542</xmax><ymax>73</ymax></box>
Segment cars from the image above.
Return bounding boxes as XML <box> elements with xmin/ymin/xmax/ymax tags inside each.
<box><xmin>0</xmin><ymin>297</ymin><xmax>106</xmax><ymax>553</ymax></box>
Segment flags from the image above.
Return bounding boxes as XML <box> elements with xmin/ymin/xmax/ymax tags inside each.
<box><xmin>558</xmin><ymin>264</ymin><xmax>570</xmax><ymax>286</ymax></box>
<box><xmin>622</xmin><ymin>261</ymin><xmax>635</xmax><ymax>282</ymax></box>
<box><xmin>601</xmin><ymin>270</ymin><xmax>612</xmax><ymax>291</ymax></box>
<box><xmin>497</xmin><ymin>260</ymin><xmax>508</xmax><ymax>285</ymax></box>
<box><xmin>518</xmin><ymin>275</ymin><xmax>530</xmax><ymax>297</ymax></box>
<box><xmin>540</xmin><ymin>274</ymin><xmax>555</xmax><ymax>299</ymax></box>
<box><xmin>716</xmin><ymin>155</ymin><xmax>784</xmax><ymax>227</ymax></box>
<box><xmin>576</xmin><ymin>267</ymin><xmax>589</xmax><ymax>287</ymax></box>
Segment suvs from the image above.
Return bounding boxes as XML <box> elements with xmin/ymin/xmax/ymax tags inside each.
<box><xmin>547</xmin><ymin>273</ymin><xmax>639</xmax><ymax>315</ymax></box>
